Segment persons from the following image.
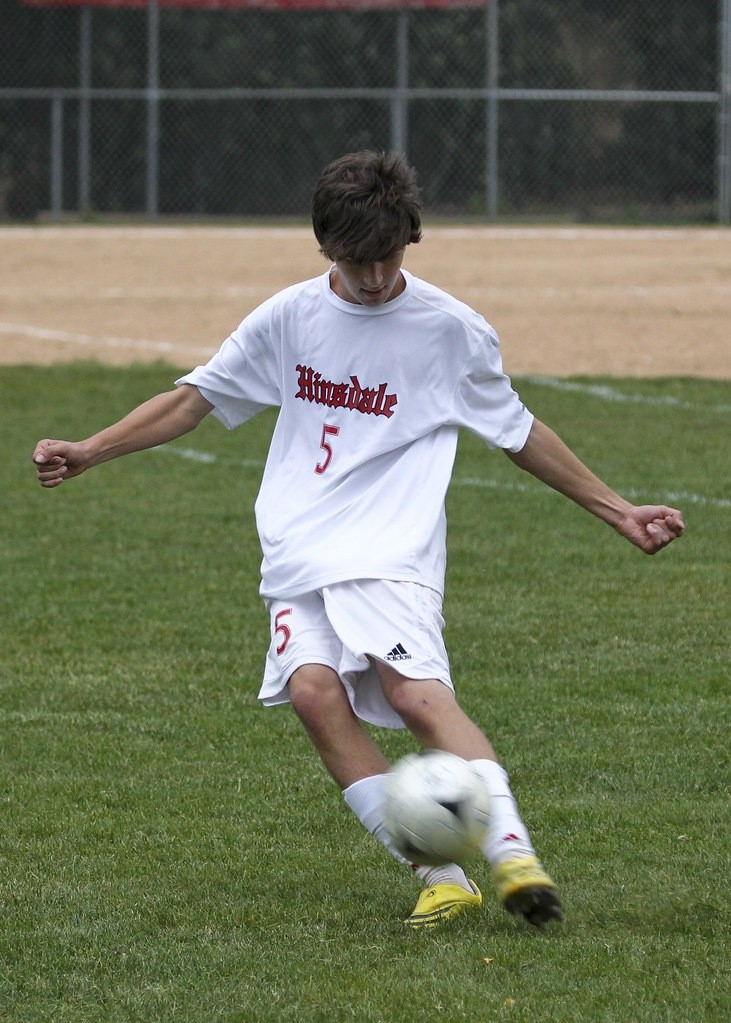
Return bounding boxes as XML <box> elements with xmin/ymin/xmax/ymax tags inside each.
<box><xmin>32</xmin><ymin>151</ymin><xmax>684</xmax><ymax>931</ymax></box>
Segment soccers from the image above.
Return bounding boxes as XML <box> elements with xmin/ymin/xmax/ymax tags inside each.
<box><xmin>377</xmin><ymin>747</ymin><xmax>495</xmax><ymax>868</ymax></box>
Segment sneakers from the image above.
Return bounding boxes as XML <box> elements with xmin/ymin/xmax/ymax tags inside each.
<box><xmin>403</xmin><ymin>879</ymin><xmax>483</xmax><ymax>929</ymax></box>
<box><xmin>491</xmin><ymin>855</ymin><xmax>565</xmax><ymax>931</ymax></box>
